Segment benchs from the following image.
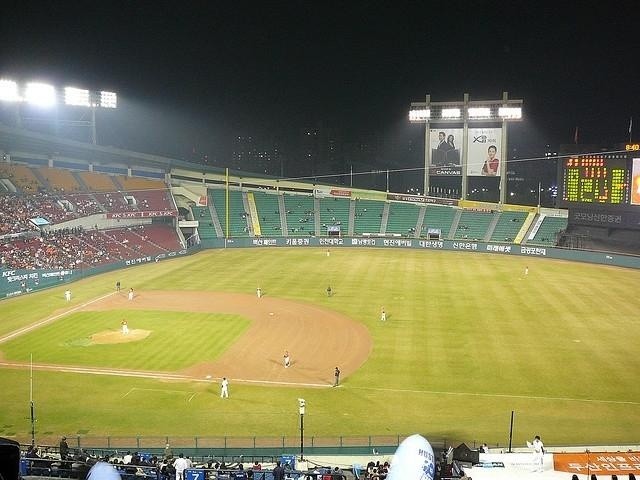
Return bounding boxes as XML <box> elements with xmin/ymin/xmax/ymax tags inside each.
<box><xmin>14</xmin><ymin>443</ymin><xmax>395</xmax><ymax>480</ymax></box>
<box><xmin>0</xmin><ymin>161</ymin><xmax>186</xmax><ymax>277</ymax></box>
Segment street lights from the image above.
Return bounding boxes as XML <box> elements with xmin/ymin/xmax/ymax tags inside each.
<box><xmin>0</xmin><ymin>77</ymin><xmax>56</xmax><ymax>128</ymax></box>
<box><xmin>63</xmin><ymin>85</ymin><xmax>118</xmax><ymax>144</ymax></box>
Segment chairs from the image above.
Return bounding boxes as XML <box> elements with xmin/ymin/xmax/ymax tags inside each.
<box><xmin>353</xmin><ymin>199</ymin><xmax>567</xmax><ymax>248</ymax></box>
<box><xmin>190</xmin><ymin>188</ymin><xmax>350</xmax><ymax>240</ymax></box>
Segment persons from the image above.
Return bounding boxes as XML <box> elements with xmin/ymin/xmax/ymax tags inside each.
<box><xmin>325</xmin><ymin>285</ymin><xmax>332</xmax><ymax>297</ymax></box>
<box><xmin>0</xmin><ymin>159</ymin><xmax>153</xmax><ymax>274</ymax></box>
<box><xmin>526</xmin><ymin>435</ymin><xmax>545</xmax><ymax>473</ymax></box>
<box><xmin>524</xmin><ymin>266</ymin><xmax>529</xmax><ymax>279</ymax></box>
<box><xmin>481</xmin><ymin>144</ymin><xmax>499</xmax><ymax>177</ymax></box>
<box><xmin>115</xmin><ymin>279</ymin><xmax>121</xmax><ymax>293</ymax></box>
<box><xmin>332</xmin><ymin>366</ymin><xmax>340</xmax><ymax>388</ymax></box>
<box><xmin>283</xmin><ymin>349</ymin><xmax>291</xmax><ymax>368</ymax></box>
<box><xmin>26</xmin><ymin>287</ymin><xmax>32</xmax><ymax>293</ymax></box>
<box><xmin>380</xmin><ymin>306</ymin><xmax>386</xmax><ymax>322</ymax></box>
<box><xmin>433</xmin><ymin>131</ymin><xmax>450</xmax><ymax>169</ymax></box>
<box><xmin>198</xmin><ymin>192</ymin><xmax>367</xmax><ymax>240</ymax></box>
<box><xmin>256</xmin><ymin>287</ymin><xmax>262</xmax><ymax>298</ymax></box>
<box><xmin>21</xmin><ymin>282</ymin><xmax>26</xmax><ymax>293</ymax></box>
<box><xmin>445</xmin><ymin>134</ymin><xmax>457</xmax><ymax>165</ymax></box>
<box><xmin>65</xmin><ymin>288</ymin><xmax>71</xmax><ymax>301</ymax></box>
<box><xmin>326</xmin><ymin>248</ymin><xmax>330</xmax><ymax>257</ymax></box>
<box><xmin>127</xmin><ymin>288</ymin><xmax>134</xmax><ymax>302</ymax></box>
<box><xmin>219</xmin><ymin>377</ymin><xmax>229</xmax><ymax>399</ymax></box>
<box><xmin>120</xmin><ymin>318</ymin><xmax>129</xmax><ymax>335</ymax></box>
